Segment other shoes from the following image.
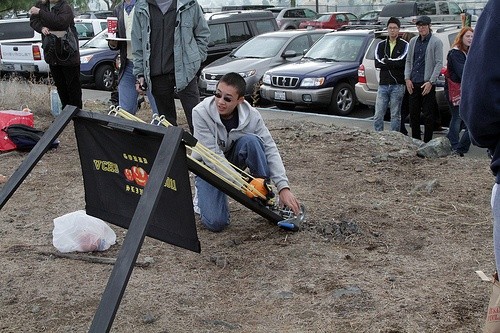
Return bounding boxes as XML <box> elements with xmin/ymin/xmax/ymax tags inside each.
<box><xmin>193</xmin><ymin>180</ymin><xmax>200</xmax><ymax>214</ymax></box>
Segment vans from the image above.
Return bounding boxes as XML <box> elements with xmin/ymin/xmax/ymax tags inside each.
<box><xmin>377</xmin><ymin>0</ymin><xmax>479</xmax><ymax>25</ymax></box>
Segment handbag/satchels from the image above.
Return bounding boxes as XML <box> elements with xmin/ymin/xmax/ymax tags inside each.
<box><xmin>447</xmin><ymin>78</ymin><xmax>461</xmax><ymax>106</ymax></box>
<box><xmin>41</xmin><ymin>29</ymin><xmax>77</xmax><ymax>64</ymax></box>
<box><xmin>0</xmin><ymin>104</ymin><xmax>34</xmax><ymax>152</ymax></box>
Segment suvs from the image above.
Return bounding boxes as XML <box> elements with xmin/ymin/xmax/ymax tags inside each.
<box><xmin>195</xmin><ymin>8</ymin><xmax>279</xmax><ymax>79</ymax></box>
<box><xmin>354</xmin><ymin>21</ymin><xmax>477</xmax><ymax>122</ymax></box>
<box><xmin>0</xmin><ymin>9</ymin><xmax>122</xmax><ymax>86</ymax></box>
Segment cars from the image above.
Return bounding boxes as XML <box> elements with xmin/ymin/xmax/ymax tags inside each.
<box><xmin>347</xmin><ymin>9</ymin><xmax>380</xmax><ymax>30</ymax></box>
<box><xmin>298</xmin><ymin>11</ymin><xmax>359</xmax><ymax>31</ymax></box>
<box><xmin>198</xmin><ymin>27</ymin><xmax>340</xmax><ymax>107</ymax></box>
<box><xmin>259</xmin><ymin>19</ymin><xmax>388</xmax><ymax>117</ymax></box>
<box><xmin>221</xmin><ymin>4</ymin><xmax>318</xmax><ymax>31</ymax></box>
<box><xmin>79</xmin><ymin>27</ymin><xmax>122</xmax><ymax>91</ymax></box>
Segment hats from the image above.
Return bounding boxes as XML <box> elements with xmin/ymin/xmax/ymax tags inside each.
<box><xmin>415</xmin><ymin>16</ymin><xmax>431</xmax><ymax>25</ymax></box>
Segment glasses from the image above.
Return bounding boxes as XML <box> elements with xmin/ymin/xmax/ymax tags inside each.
<box><xmin>212</xmin><ymin>91</ymin><xmax>238</xmax><ymax>102</ymax></box>
<box><xmin>388</xmin><ymin>26</ymin><xmax>400</xmax><ymax>29</ymax></box>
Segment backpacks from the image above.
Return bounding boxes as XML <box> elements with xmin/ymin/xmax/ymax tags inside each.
<box><xmin>1</xmin><ymin>124</ymin><xmax>59</xmax><ymax>153</ymax></box>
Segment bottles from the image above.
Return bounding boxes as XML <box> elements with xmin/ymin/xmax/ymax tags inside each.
<box><xmin>50</xmin><ymin>86</ymin><xmax>60</xmax><ymax>115</ymax></box>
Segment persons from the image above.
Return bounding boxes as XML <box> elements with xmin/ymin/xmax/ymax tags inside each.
<box><xmin>131</xmin><ymin>0</ymin><xmax>211</xmax><ymax>131</ymax></box>
<box><xmin>405</xmin><ymin>16</ymin><xmax>443</xmax><ymax>142</ymax></box>
<box><xmin>30</xmin><ymin>0</ymin><xmax>82</xmax><ymax>109</ymax></box>
<box><xmin>458</xmin><ymin>0</ymin><xmax>500</xmax><ymax>276</ymax></box>
<box><xmin>444</xmin><ymin>27</ymin><xmax>474</xmax><ymax>156</ymax></box>
<box><xmin>190</xmin><ymin>72</ymin><xmax>301</xmax><ymax>231</ymax></box>
<box><xmin>108</xmin><ymin>0</ymin><xmax>159</xmax><ymax>125</ymax></box>
<box><xmin>373</xmin><ymin>17</ymin><xmax>409</xmax><ymax>131</ymax></box>
